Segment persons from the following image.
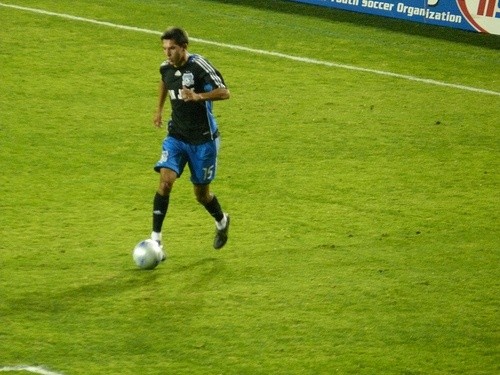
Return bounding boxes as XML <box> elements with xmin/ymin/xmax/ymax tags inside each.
<box><xmin>151</xmin><ymin>28</ymin><xmax>230</xmax><ymax>260</ymax></box>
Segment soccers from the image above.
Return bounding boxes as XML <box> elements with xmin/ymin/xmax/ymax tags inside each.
<box><xmin>133</xmin><ymin>239</ymin><xmax>163</xmax><ymax>269</ymax></box>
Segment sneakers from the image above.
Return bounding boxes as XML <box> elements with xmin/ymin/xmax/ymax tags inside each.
<box><xmin>213</xmin><ymin>215</ymin><xmax>231</xmax><ymax>249</ymax></box>
